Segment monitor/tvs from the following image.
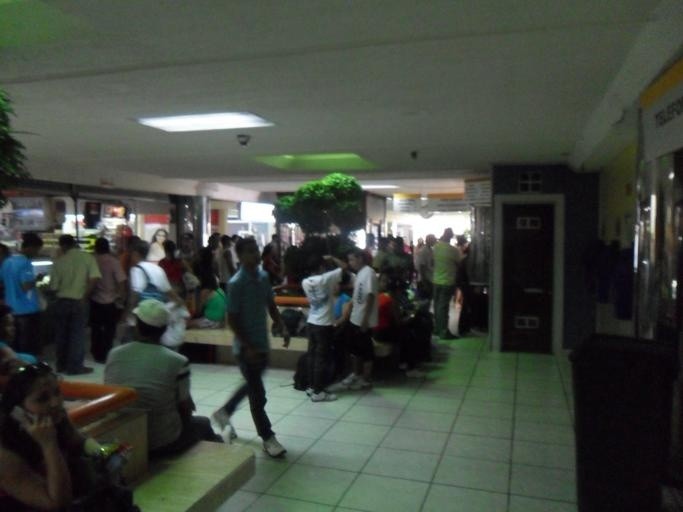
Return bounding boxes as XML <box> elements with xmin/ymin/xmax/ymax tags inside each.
<box><xmin>82</xmin><ymin>199</ymin><xmax>129</xmax><ymax>229</ymax></box>
<box><xmin>10</xmin><ymin>196</ymin><xmax>65</xmax><ymax>233</ymax></box>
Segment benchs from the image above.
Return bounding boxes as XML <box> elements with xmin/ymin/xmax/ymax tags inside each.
<box><xmin>126</xmin><ymin>435</ymin><xmax>262</xmax><ymax>510</ymax></box>
<box><xmin>180</xmin><ymin>327</ymin><xmax>309</xmax><ymax>355</ymax></box>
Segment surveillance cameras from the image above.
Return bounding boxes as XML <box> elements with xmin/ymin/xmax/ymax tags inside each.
<box><xmin>236</xmin><ymin>134</ymin><xmax>249</xmax><ymax>146</ymax></box>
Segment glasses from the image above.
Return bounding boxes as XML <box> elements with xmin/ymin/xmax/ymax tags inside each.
<box><xmin>12</xmin><ymin>361</ymin><xmax>51</xmax><ymax>377</ymax></box>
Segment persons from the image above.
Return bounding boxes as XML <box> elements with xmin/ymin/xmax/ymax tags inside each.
<box><xmin>262</xmin><ymin>227</ymin><xmax>475</xmax><ymax>402</ymax></box>
<box><xmin>208</xmin><ymin>235</ymin><xmax>293</xmax><ymax>458</ymax></box>
<box><xmin>0</xmin><ymin>361</ymin><xmax>141</xmax><ymax>512</ymax></box>
<box><xmin>86</xmin><ymin>233</ymin><xmax>241</xmax><ymax>365</ymax></box>
<box><xmin>102</xmin><ymin>298</ymin><xmax>234</xmax><ymax>456</ymax></box>
<box><xmin>46</xmin><ymin>235</ymin><xmax>94</xmax><ymax>376</ymax></box>
<box><xmin>146</xmin><ymin>228</ymin><xmax>168</xmax><ymax>261</ymax></box>
<box><xmin>1</xmin><ymin>231</ymin><xmax>45</xmax><ymax>356</ymax></box>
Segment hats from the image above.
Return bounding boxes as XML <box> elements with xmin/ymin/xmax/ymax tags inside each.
<box><xmin>130</xmin><ymin>297</ymin><xmax>173</xmax><ymax>328</ymax></box>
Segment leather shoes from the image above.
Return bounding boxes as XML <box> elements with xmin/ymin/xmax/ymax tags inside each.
<box><xmin>68</xmin><ymin>366</ymin><xmax>95</xmax><ymax>375</ymax></box>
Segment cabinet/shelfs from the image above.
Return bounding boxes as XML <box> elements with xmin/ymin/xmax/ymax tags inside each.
<box><xmin>568</xmin><ymin>329</ymin><xmax>678</xmax><ymax>509</ymax></box>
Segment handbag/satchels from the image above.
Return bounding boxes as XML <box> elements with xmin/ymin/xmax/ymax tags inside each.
<box><xmin>139</xmin><ymin>286</ymin><xmax>168</xmax><ymax>304</ymax></box>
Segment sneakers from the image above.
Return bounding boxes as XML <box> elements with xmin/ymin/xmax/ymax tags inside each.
<box><xmin>311</xmin><ymin>391</ymin><xmax>338</xmax><ymax>402</ymax></box>
<box><xmin>349</xmin><ymin>376</ymin><xmax>373</xmax><ymax>390</ymax></box>
<box><xmin>213</xmin><ymin>408</ymin><xmax>237</xmax><ymax>440</ymax></box>
<box><xmin>342</xmin><ymin>371</ymin><xmax>357</xmax><ymax>384</ymax></box>
<box><xmin>305</xmin><ymin>385</ymin><xmax>315</xmax><ymax>395</ymax></box>
<box><xmin>263</xmin><ymin>435</ymin><xmax>287</xmax><ymax>457</ymax></box>
